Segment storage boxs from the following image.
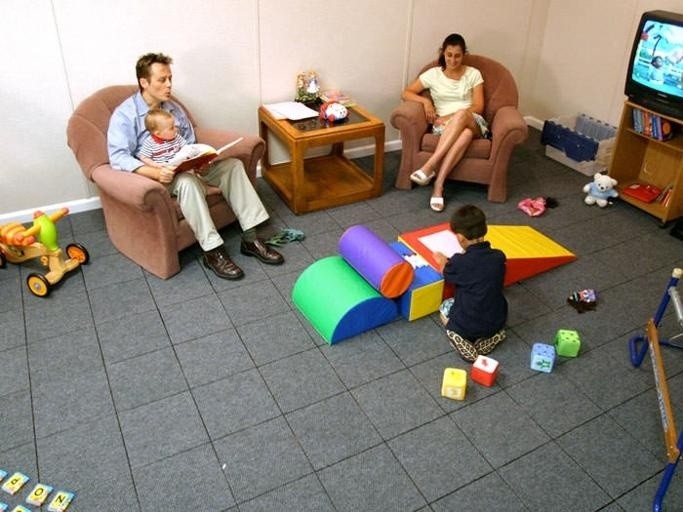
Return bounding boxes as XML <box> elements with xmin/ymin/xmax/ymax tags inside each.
<box><xmin>540</xmin><ymin>111</ymin><xmax>616</xmax><ymax>176</ymax></box>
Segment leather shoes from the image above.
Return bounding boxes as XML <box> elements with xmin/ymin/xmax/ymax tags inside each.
<box><xmin>240</xmin><ymin>237</ymin><xmax>284</xmax><ymax>264</ymax></box>
<box><xmin>203</xmin><ymin>247</ymin><xmax>243</xmax><ymax>279</ymax></box>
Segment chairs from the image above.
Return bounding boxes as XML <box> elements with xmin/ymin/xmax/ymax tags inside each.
<box><xmin>64</xmin><ymin>82</ymin><xmax>267</xmax><ymax>279</ymax></box>
<box><xmin>388</xmin><ymin>54</ymin><xmax>529</xmax><ymax>203</ymax></box>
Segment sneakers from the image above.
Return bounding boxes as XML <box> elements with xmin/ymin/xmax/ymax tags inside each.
<box><xmin>474</xmin><ymin>330</ymin><xmax>506</xmax><ymax>354</ymax></box>
<box><xmin>445</xmin><ymin>327</ymin><xmax>479</xmax><ymax>362</ymax></box>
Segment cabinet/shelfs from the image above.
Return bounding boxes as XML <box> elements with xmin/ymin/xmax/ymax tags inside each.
<box><xmin>604</xmin><ymin>99</ymin><xmax>682</xmax><ymax>229</ymax></box>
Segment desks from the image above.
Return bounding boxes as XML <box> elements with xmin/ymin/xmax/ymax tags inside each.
<box><xmin>256</xmin><ymin>95</ymin><xmax>384</xmax><ymax>215</ymax></box>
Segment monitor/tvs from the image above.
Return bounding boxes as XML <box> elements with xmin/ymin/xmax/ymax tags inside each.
<box><xmin>623</xmin><ymin>9</ymin><xmax>683</xmax><ymax>120</ymax></box>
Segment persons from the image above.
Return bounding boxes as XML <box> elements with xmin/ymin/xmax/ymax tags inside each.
<box><xmin>402</xmin><ymin>34</ymin><xmax>485</xmax><ymax>212</ymax></box>
<box><xmin>137</xmin><ymin>107</ymin><xmax>225</xmax><ymax>201</ymax></box>
<box><xmin>107</xmin><ymin>52</ymin><xmax>284</xmax><ymax>280</ymax></box>
<box><xmin>440</xmin><ymin>203</ymin><xmax>508</xmax><ymax>362</ymax></box>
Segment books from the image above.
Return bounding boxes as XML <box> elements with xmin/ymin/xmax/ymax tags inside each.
<box><xmin>630</xmin><ymin>106</ymin><xmax>671</xmax><ymax>143</ymax></box>
<box><xmin>168</xmin><ymin>134</ymin><xmax>246</xmax><ymax>174</ymax></box>
<box><xmin>657</xmin><ymin>175</ymin><xmax>674</xmax><ymax>212</ymax></box>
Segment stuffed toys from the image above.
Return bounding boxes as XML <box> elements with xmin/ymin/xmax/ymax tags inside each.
<box><xmin>581</xmin><ymin>171</ymin><xmax>619</xmax><ymax>211</ymax></box>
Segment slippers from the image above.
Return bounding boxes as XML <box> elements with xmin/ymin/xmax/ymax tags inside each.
<box><xmin>409</xmin><ymin>168</ymin><xmax>436</xmax><ymax>187</ymax></box>
<box><xmin>430</xmin><ymin>191</ymin><xmax>446</xmax><ymax>212</ymax></box>
